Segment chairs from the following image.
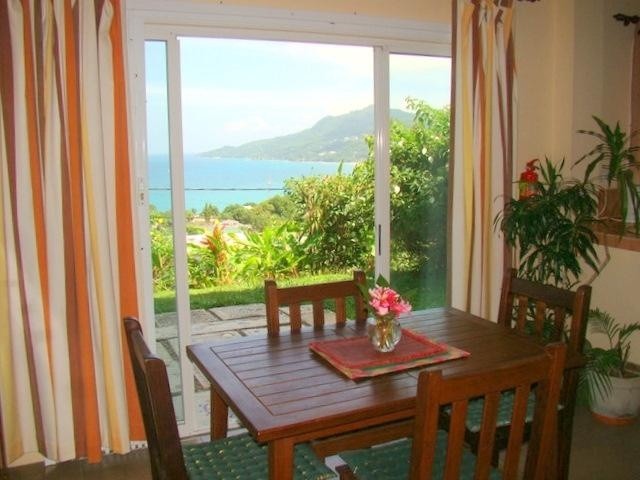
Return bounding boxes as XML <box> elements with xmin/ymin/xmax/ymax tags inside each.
<box><xmin>123</xmin><ymin>316</ymin><xmax>355</xmax><ymax>480</ymax></box>
<box><xmin>329</xmin><ymin>341</ymin><xmax>567</xmax><ymax>480</ymax></box>
<box><xmin>437</xmin><ymin>268</ymin><xmax>591</xmax><ymax>480</ymax></box>
<box><xmin>262</xmin><ymin>270</ymin><xmax>367</xmax><ymax>340</ymax></box>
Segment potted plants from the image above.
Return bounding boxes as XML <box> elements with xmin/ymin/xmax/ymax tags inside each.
<box><xmin>585</xmin><ymin>306</ymin><xmax>640</xmax><ymax>425</ymax></box>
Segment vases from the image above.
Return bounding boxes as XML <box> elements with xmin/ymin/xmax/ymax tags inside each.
<box><xmin>367</xmin><ymin>314</ymin><xmax>401</xmax><ymax>353</ymax></box>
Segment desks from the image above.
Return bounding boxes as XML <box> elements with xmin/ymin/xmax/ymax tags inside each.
<box><xmin>187</xmin><ymin>302</ymin><xmax>586</xmax><ymax>480</ymax></box>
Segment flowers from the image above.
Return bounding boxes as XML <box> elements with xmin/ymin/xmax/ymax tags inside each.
<box><xmin>355</xmin><ymin>273</ymin><xmax>412</xmax><ymax>347</ymax></box>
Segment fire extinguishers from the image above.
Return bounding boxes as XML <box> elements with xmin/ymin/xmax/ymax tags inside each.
<box><xmin>518</xmin><ymin>159</ymin><xmax>540</xmax><ymax>204</ymax></box>
